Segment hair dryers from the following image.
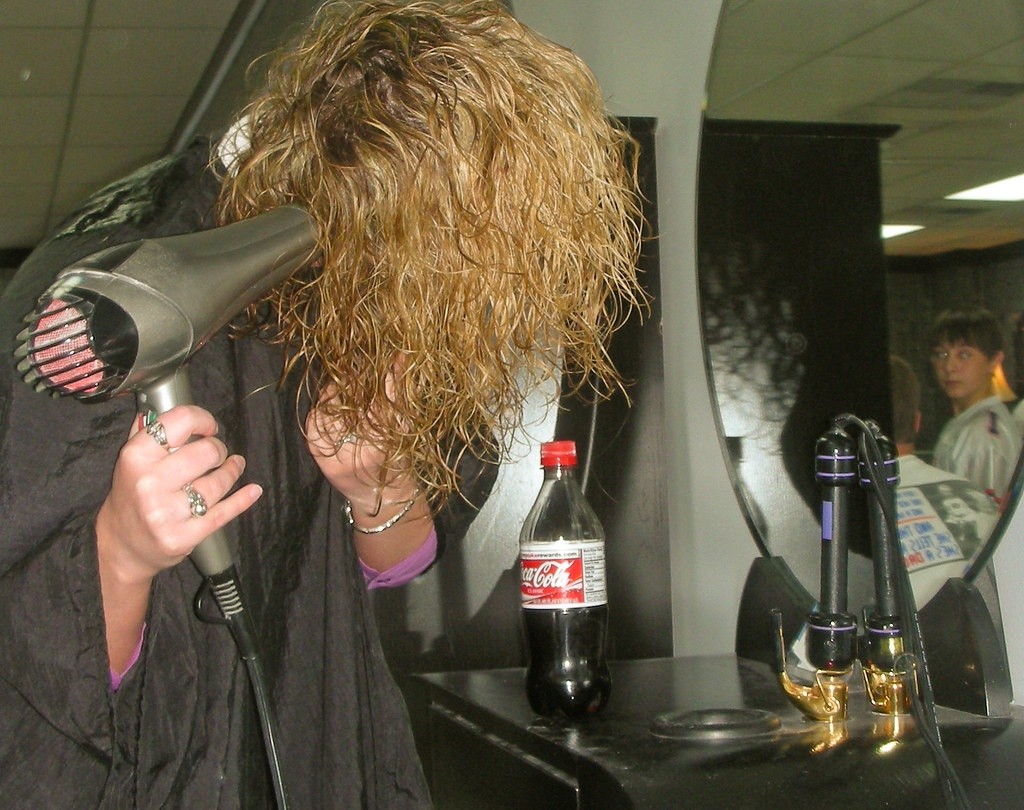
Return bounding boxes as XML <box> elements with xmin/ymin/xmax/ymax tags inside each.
<box><xmin>16</xmin><ymin>205</ymin><xmax>321</xmax><ymax>625</ymax></box>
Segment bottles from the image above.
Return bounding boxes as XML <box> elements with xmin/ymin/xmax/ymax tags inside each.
<box><xmin>519</xmin><ymin>442</ymin><xmax>610</xmax><ymax>719</ymax></box>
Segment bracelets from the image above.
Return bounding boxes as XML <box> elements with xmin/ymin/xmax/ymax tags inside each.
<box><xmin>340</xmin><ymin>485</ymin><xmax>423</xmax><ymax>534</ymax></box>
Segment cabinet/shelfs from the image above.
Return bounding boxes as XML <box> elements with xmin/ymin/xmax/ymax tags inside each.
<box><xmin>695</xmin><ymin>119</ymin><xmax>902</xmax><ymax>621</ymax></box>
<box><xmin>404</xmin><ymin>655</ymin><xmax>1024</xmax><ymax>810</ymax></box>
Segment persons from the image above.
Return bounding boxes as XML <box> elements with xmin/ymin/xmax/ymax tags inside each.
<box><xmin>923</xmin><ymin>307</ymin><xmax>1022</xmax><ymax>503</ymax></box>
<box><xmin>1</xmin><ymin>1</ymin><xmax>654</xmax><ymax>810</ymax></box>
<box><xmin>887</xmin><ymin>354</ymin><xmax>1001</xmax><ymax>619</ymax></box>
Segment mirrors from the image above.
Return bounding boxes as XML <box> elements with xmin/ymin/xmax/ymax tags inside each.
<box><xmin>694</xmin><ymin>0</ymin><xmax>1024</xmax><ymax>635</ymax></box>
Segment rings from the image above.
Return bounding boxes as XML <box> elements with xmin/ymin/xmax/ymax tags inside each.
<box><xmin>183</xmin><ymin>484</ymin><xmax>207</xmax><ymax>517</ymax></box>
<box><xmin>145</xmin><ymin>421</ymin><xmax>168</xmax><ymax>448</ymax></box>
<box><xmin>332</xmin><ymin>433</ymin><xmax>355</xmax><ymax>445</ymax></box>
